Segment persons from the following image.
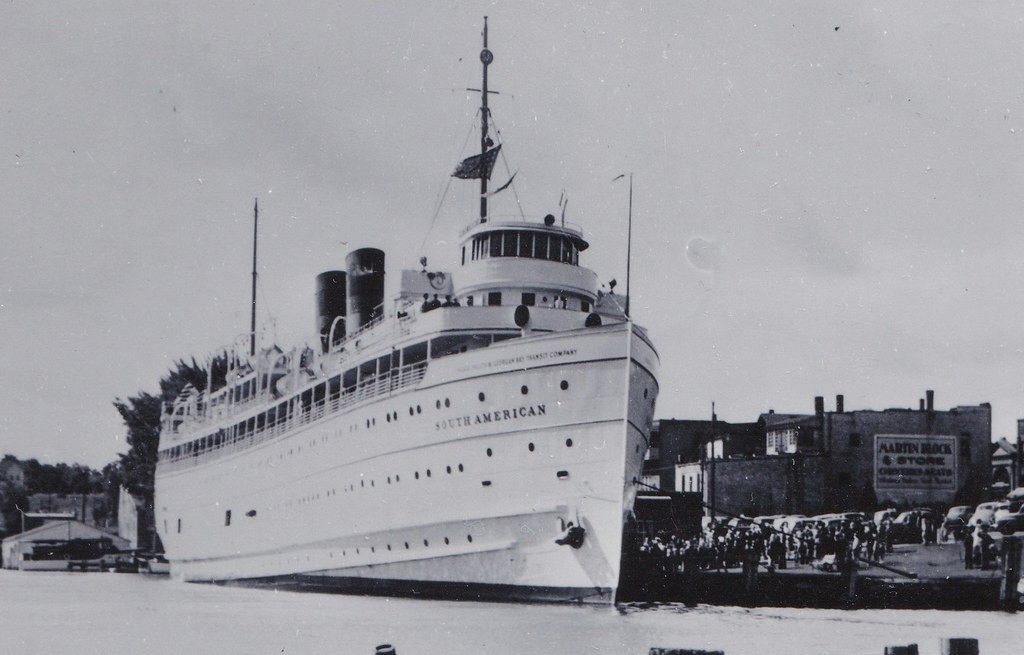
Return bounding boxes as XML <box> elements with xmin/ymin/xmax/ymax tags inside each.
<box><xmin>960</xmin><ymin>518</ymin><xmax>1002</xmax><ymax>569</ymax></box>
<box><xmin>632</xmin><ymin>510</ymin><xmax>897</xmax><ymax>569</ymax></box>
<box><xmin>422</xmin><ymin>292</ymin><xmax>460</xmax><ymax>313</ymax></box>
<box><xmin>541</xmin><ymin>293</ymin><xmax>565</xmax><ymax>308</ymax></box>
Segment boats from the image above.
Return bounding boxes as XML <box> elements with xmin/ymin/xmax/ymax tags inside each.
<box><xmin>20</xmin><ymin>535</ymin><xmax>133</xmax><ymax>571</ymax></box>
<box><xmin>154</xmin><ymin>15</ymin><xmax>662</xmax><ymax>602</ymax></box>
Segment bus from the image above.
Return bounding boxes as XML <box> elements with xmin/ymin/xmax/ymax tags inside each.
<box><xmin>704</xmin><ymin>486</ymin><xmax>1024</xmax><ymax>554</ymax></box>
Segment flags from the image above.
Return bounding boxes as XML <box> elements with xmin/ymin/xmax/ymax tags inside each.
<box><xmin>450</xmin><ymin>145</ymin><xmax>502</xmax><ymax>180</ymax></box>
